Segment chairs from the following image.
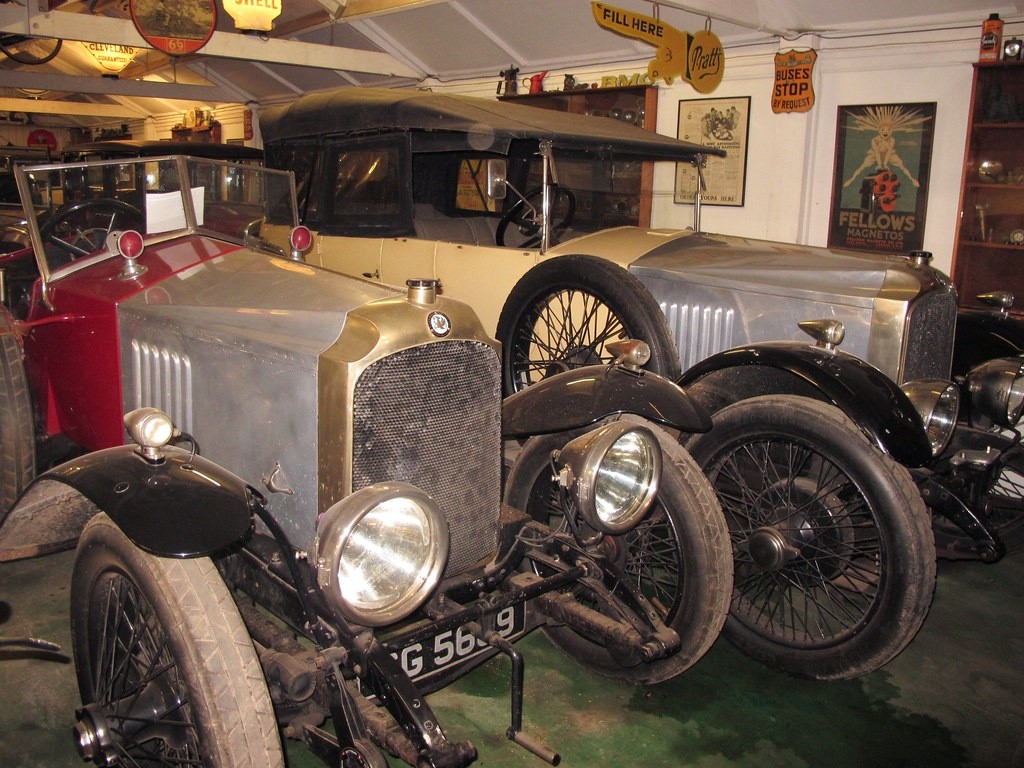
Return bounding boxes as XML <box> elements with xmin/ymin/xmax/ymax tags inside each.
<box><xmin>336</xmin><ymin>200</ymin><xmax>592</xmax><ymax>247</ymax></box>
<box><xmin>44</xmin><ymin>244</ymin><xmax>81</xmax><ymax>273</ymax></box>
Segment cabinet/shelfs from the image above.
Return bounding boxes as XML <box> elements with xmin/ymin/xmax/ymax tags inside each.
<box><xmin>949</xmin><ymin>58</ymin><xmax>1024</xmax><ymax>326</ymax></box>
<box><xmin>496</xmin><ymin>84</ymin><xmax>660</xmax><ymax>228</ymax></box>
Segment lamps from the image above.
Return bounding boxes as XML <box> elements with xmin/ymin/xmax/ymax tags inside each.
<box><xmin>76</xmin><ymin>41</ymin><xmax>142</xmax><ymax>81</ymax></box>
<box><xmin>221</xmin><ymin>0</ymin><xmax>283</xmax><ymax>42</ymax></box>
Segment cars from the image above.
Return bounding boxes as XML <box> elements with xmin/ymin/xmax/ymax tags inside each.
<box><xmin>1</xmin><ymin>85</ymin><xmax>1024</xmax><ymax>767</ymax></box>
